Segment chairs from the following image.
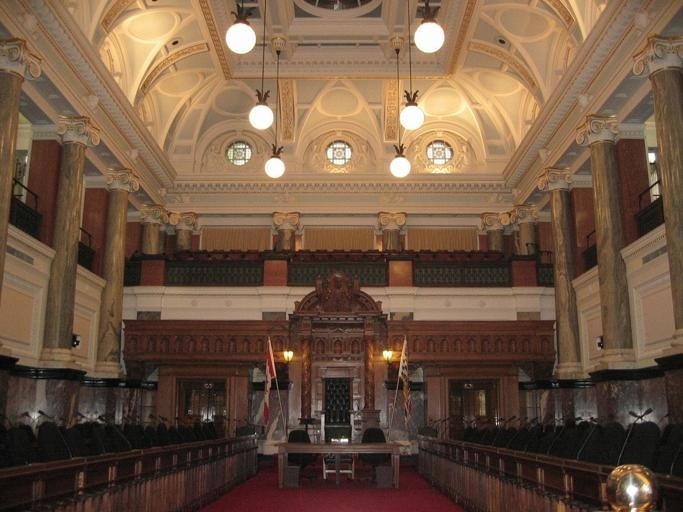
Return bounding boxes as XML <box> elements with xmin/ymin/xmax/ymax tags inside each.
<box><xmin>417</xmin><ymin>422</ymin><xmax>683</xmax><ymax>478</ymax></box>
<box><xmin>284</xmin><ymin>430</ymin><xmax>313</xmax><ymax>488</ymax></box>
<box><xmin>362</xmin><ymin>427</ymin><xmax>392</xmax><ymax>482</ymax></box>
<box><xmin>0</xmin><ymin>420</ymin><xmax>254</xmax><ymax>468</ymax></box>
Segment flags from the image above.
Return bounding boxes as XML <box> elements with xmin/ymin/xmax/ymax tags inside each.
<box><xmin>262</xmin><ymin>339</ymin><xmax>278</xmax><ymax>436</ymax></box>
<box><xmin>397</xmin><ymin>339</ymin><xmax>414</xmax><ymax>427</ymax></box>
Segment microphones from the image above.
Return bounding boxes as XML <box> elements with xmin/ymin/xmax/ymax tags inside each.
<box><xmin>422</xmin><ymin>408</ymin><xmax>683</xmax><ymax>477</ymax></box>
<box><xmin>0</xmin><ymin>408</ymin><xmax>255</xmax><ymax>468</ymax></box>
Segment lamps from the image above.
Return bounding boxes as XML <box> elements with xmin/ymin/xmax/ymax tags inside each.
<box><xmin>389</xmin><ymin>36</ymin><xmax>411</xmax><ymax>179</ymax></box>
<box><xmin>400</xmin><ymin>0</ymin><xmax>424</xmax><ymax>131</ymax></box>
<box><xmin>265</xmin><ymin>36</ymin><xmax>288</xmax><ymax>179</ymax></box>
<box><xmin>283</xmin><ymin>322</ymin><xmax>298</xmax><ymax>364</ymax></box>
<box><xmin>382</xmin><ymin>319</ymin><xmax>393</xmax><ymax>363</ymax></box>
<box><xmin>248</xmin><ymin>0</ymin><xmax>274</xmax><ymax>131</ymax></box>
<box><xmin>414</xmin><ymin>0</ymin><xmax>445</xmax><ymax>53</ymax></box>
<box><xmin>225</xmin><ymin>0</ymin><xmax>256</xmax><ymax>55</ymax></box>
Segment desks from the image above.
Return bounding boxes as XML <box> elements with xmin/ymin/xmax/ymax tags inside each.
<box><xmin>274</xmin><ymin>442</ymin><xmax>411</xmax><ymax>489</ymax></box>
